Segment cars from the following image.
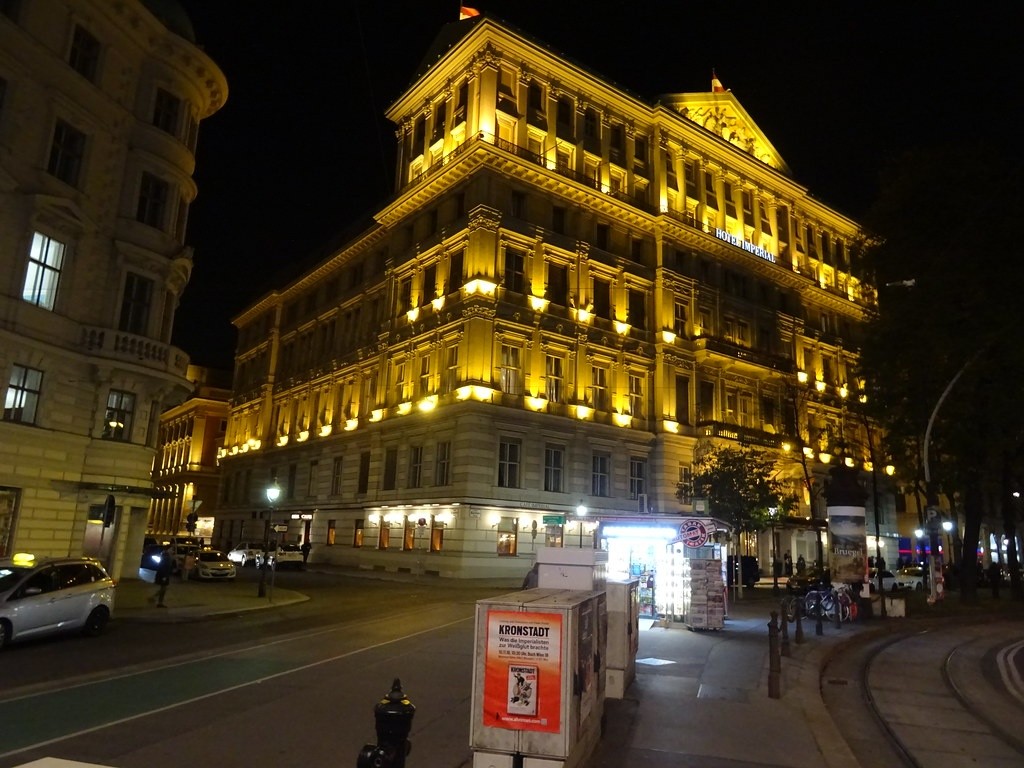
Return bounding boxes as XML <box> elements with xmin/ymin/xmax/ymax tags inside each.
<box><xmin>255</xmin><ymin>540</ymin><xmax>305</xmax><ymax>571</ymax></box>
<box><xmin>1001</xmin><ymin>561</ymin><xmax>1024</xmax><ymax>589</ymax></box>
<box><xmin>227</xmin><ymin>540</ymin><xmax>269</xmax><ymax>567</ymax></box>
<box><xmin>141</xmin><ymin>543</ymin><xmax>187</xmax><ymax>576</ymax></box>
<box><xmin>170</xmin><ymin>544</ymin><xmax>204</xmax><ymax>564</ymax></box>
<box><xmin>896</xmin><ymin>565</ymin><xmax>944</xmax><ymax>594</ymax></box>
<box><xmin>727</xmin><ymin>554</ymin><xmax>760</xmax><ymax>589</ymax></box>
<box><xmin>869</xmin><ymin>567</ymin><xmax>900</xmax><ymax>595</ymax></box>
<box><xmin>786</xmin><ymin>565</ymin><xmax>831</xmax><ymax>596</ymax></box>
<box><xmin>0</xmin><ymin>556</ymin><xmax>118</xmax><ymax>652</ymax></box>
<box><xmin>943</xmin><ymin>560</ymin><xmax>986</xmax><ymax>592</ymax></box>
<box><xmin>184</xmin><ymin>547</ymin><xmax>237</xmax><ymax>582</ymax></box>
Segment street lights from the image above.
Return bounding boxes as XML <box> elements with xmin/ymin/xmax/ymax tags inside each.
<box><xmin>257</xmin><ymin>476</ymin><xmax>283</xmax><ymax>597</ymax></box>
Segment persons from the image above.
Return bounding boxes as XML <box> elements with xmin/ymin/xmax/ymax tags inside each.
<box><xmin>148</xmin><ymin>551</ymin><xmax>171</xmax><ymax>608</ymax></box>
<box><xmin>522</xmin><ymin>562</ymin><xmax>538</xmax><ymax>590</ymax></box>
<box><xmin>868</xmin><ymin>555</ymin><xmax>919</xmax><ymax>571</ymax></box>
<box><xmin>784</xmin><ymin>549</ymin><xmax>806</xmax><ymax>578</ymax></box>
<box><xmin>302</xmin><ymin>539</ymin><xmax>311</xmax><ymax>564</ymax></box>
<box><xmin>199</xmin><ymin>537</ymin><xmax>205</xmax><ymax>549</ymax></box>
<box><xmin>991</xmin><ymin>561</ymin><xmax>1002</xmax><ymax>598</ymax></box>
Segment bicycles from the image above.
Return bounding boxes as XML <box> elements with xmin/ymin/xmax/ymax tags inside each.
<box><xmin>787</xmin><ymin>580</ymin><xmax>857</xmax><ymax>624</ymax></box>
